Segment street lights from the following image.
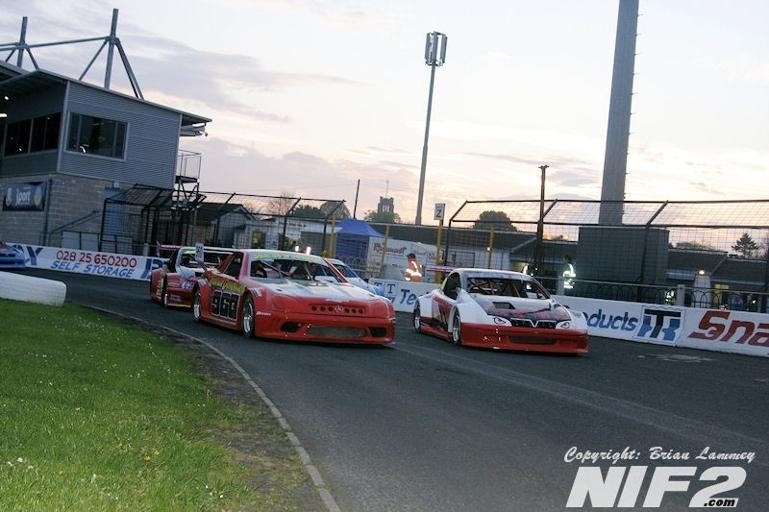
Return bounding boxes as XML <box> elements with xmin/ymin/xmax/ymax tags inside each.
<box><xmin>537</xmin><ymin>164</ymin><xmax>550</xmax><ymax>283</ymax></box>
<box><xmin>409</xmin><ymin>30</ymin><xmax>449</xmax><ymax>224</ymax></box>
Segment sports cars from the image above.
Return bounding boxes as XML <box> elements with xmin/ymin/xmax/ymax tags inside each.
<box><xmin>410</xmin><ymin>264</ymin><xmax>591</xmax><ymax>357</ymax></box>
<box><xmin>148</xmin><ymin>243</ymin><xmax>396</xmax><ymax>348</ymax></box>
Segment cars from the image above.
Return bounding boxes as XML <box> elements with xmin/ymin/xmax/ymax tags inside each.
<box><xmin>1</xmin><ymin>239</ymin><xmax>27</xmax><ymax>271</ymax></box>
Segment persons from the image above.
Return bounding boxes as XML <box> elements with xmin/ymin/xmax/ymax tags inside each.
<box><xmin>288</xmin><ymin>240</ymin><xmax>297</xmax><ymax>251</ymax></box>
<box><xmin>401</xmin><ymin>254</ymin><xmax>423</xmax><ymax>282</ymax></box>
<box><xmin>728</xmin><ymin>292</ymin><xmax>736</xmax><ymax>310</ymax></box>
<box><xmin>748</xmin><ymin>293</ymin><xmax>756</xmax><ymax>312</ymax></box>
<box><xmin>684</xmin><ymin>287</ymin><xmax>692</xmax><ymax>307</ymax></box>
<box><xmin>732</xmin><ymin>292</ymin><xmax>744</xmax><ymax>311</ymax></box>
<box><xmin>561</xmin><ymin>254</ymin><xmax>576</xmax><ymax>295</ymax></box>
